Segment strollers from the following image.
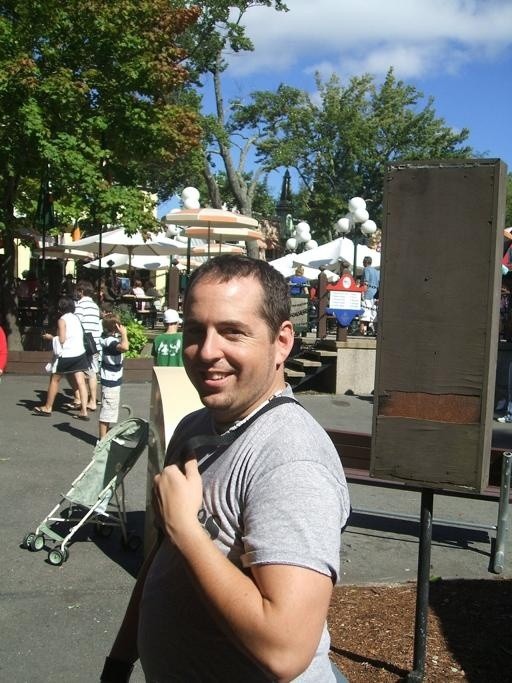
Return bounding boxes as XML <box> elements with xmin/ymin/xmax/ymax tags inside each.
<box><xmin>24</xmin><ymin>404</ymin><xmax>148</xmax><ymax>565</ymax></box>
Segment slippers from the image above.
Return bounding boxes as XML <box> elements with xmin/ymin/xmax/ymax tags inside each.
<box><xmin>31</xmin><ymin>399</ymin><xmax>101</xmax><ymax>420</ymax></box>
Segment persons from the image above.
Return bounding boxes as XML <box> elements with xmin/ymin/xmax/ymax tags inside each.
<box><xmin>96</xmin><ymin>250</ymin><xmax>355</xmax><ymax>681</ymax></box>
<box><xmin>495</xmin><ymin>269</ymin><xmax>512</xmax><ymax>423</ymax></box>
<box><xmin>94</xmin><ymin>311</ymin><xmax>130</xmax><ymax>442</ymax></box>
<box><xmin>148</xmin><ymin>306</ymin><xmax>185</xmax><ymax>368</ymax></box>
<box><xmin>58</xmin><ymin>279</ymin><xmax>104</xmax><ymax>412</ymax></box>
<box><xmin>0</xmin><ymin>268</ymin><xmax>163</xmax><ymax>329</ymax></box>
<box><xmin>32</xmin><ymin>297</ymin><xmax>91</xmax><ymax>422</ymax></box>
<box><xmin>95</xmin><ymin>300</ymin><xmax>115</xmax><ymax>406</ymax></box>
<box><xmin>287</xmin><ymin>255</ymin><xmax>380</xmax><ymax>336</ymax></box>
<box><xmin>0</xmin><ymin>322</ymin><xmax>9</xmax><ymax>376</ymax></box>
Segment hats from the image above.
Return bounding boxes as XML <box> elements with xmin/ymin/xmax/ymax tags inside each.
<box><xmin>162</xmin><ymin>308</ymin><xmax>184</xmax><ymax>325</ymax></box>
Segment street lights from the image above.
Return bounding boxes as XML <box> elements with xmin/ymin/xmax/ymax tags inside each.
<box><xmin>337</xmin><ymin>194</ymin><xmax>377</xmax><ymax>278</ymax></box>
<box><xmin>166</xmin><ymin>185</ymin><xmax>201</xmax><ymax>274</ymax></box>
<box><xmin>286</xmin><ymin>222</ymin><xmax>318</xmax><ymax>252</ymax></box>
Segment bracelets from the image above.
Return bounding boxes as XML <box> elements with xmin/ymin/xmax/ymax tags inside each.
<box><xmin>100</xmin><ymin>654</ymin><xmax>135</xmax><ymax>682</ymax></box>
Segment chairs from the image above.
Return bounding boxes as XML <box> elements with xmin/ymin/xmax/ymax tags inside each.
<box><xmin>121</xmin><ymin>295</ymin><xmax>159</xmax><ymax>329</ymax></box>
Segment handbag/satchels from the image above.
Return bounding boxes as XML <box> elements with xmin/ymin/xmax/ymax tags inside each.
<box><xmin>83</xmin><ymin>332</ymin><xmax>98</xmax><ymax>355</ymax></box>
